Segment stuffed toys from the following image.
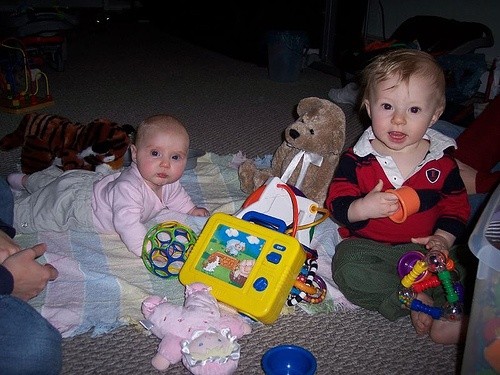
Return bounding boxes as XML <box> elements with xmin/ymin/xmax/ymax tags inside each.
<box><xmin>237</xmin><ymin>97</ymin><xmax>346</xmax><ymax>204</ymax></box>
<box><xmin>0</xmin><ymin>113</ymin><xmax>135</xmax><ymax>176</ymax></box>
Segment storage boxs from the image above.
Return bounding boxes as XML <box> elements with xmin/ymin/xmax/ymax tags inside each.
<box><xmin>459</xmin><ymin>185</ymin><xmax>500</xmax><ymax>375</ymax></box>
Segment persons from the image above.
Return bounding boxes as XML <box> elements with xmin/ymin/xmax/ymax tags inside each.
<box><xmin>5</xmin><ymin>114</ymin><xmax>210</xmax><ymax>262</ymax></box>
<box><xmin>0</xmin><ymin>174</ymin><xmax>62</xmax><ymax>375</ymax></box>
<box><xmin>141</xmin><ymin>282</ymin><xmax>251</xmax><ymax>375</ymax></box>
<box><xmin>325</xmin><ymin>49</ymin><xmax>479</xmax><ymax>344</ymax></box>
